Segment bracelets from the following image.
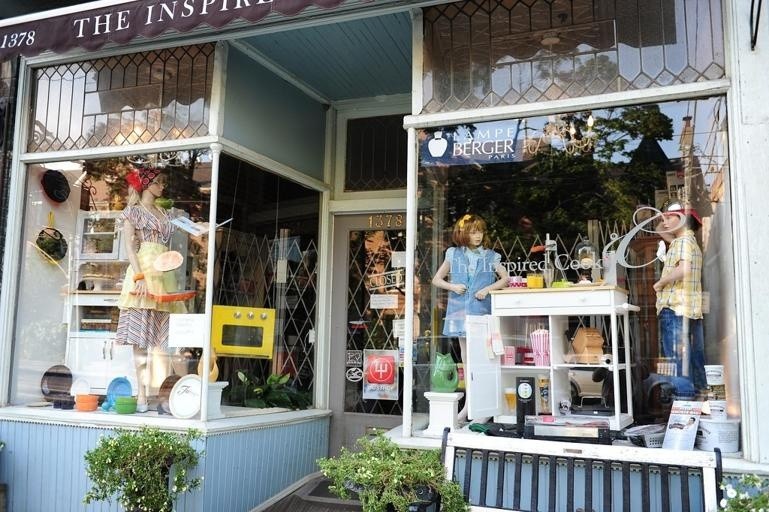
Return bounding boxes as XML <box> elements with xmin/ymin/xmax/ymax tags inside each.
<box><xmin>133</xmin><ymin>272</ymin><xmax>145</xmax><ymax>282</ymax></box>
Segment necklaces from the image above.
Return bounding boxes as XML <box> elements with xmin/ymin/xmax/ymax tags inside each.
<box><xmin>138</xmin><ymin>198</ymin><xmax>171</xmax><ymax>244</ymax></box>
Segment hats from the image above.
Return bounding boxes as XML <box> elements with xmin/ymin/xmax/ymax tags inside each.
<box><xmin>663</xmin><ymin>197</ymin><xmax>705</xmax><ymax>230</ymax></box>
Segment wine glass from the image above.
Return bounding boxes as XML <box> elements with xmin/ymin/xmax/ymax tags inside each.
<box><xmin>502</xmin><ymin>387</ymin><xmax>517</xmax><ymax>416</ymax></box>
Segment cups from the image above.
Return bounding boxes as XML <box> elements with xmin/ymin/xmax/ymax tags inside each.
<box><xmin>508</xmin><ymin>272</ymin><xmax>545</xmax><ymax>288</ymax></box>
<box><xmin>704</xmin><ymin>363</ymin><xmax>728</xmax><ymax>419</ymax></box>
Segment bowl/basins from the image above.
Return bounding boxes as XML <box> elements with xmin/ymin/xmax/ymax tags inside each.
<box><xmin>76</xmin><ymin>394</ymin><xmax>99</xmax><ymax>412</ymax></box>
<box><xmin>113</xmin><ymin>395</ymin><xmax>138</xmax><ymax>413</ymax></box>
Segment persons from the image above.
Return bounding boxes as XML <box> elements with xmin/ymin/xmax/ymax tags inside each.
<box><xmin>652</xmin><ymin>198</ymin><xmax>710</xmax><ymax>397</ymax></box>
<box><xmin>432</xmin><ymin>213</ymin><xmax>511</xmax><ymax>423</ymax></box>
<box><xmin>114</xmin><ymin>167</ymin><xmax>174</xmax><ymax>413</ymax></box>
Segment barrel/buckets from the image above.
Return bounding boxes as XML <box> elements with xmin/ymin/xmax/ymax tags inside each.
<box><xmin>697</xmin><ymin>418</ymin><xmax>741</xmax><ymax>453</ymax></box>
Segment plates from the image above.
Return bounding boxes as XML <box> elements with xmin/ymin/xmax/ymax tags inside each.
<box><xmin>157</xmin><ymin>376</ymin><xmax>180</xmax><ymax>414</ymax></box>
<box><xmin>168</xmin><ymin>373</ymin><xmax>202</xmax><ymax>418</ymax></box>
<box><xmin>40</xmin><ymin>365</ymin><xmax>74</xmax><ymax>396</ymax></box>
<box><xmin>107</xmin><ymin>377</ymin><xmax>132</xmax><ymax>408</ymax></box>
<box><xmin>72</xmin><ymin>375</ymin><xmax>91</xmax><ymax>399</ymax></box>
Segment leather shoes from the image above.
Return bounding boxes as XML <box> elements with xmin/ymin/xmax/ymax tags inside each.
<box><xmin>134</xmin><ymin>395</ymin><xmax>150</xmax><ymax>415</ymax></box>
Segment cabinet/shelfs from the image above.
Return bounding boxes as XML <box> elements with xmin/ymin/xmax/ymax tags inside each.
<box><xmin>482</xmin><ymin>284</ymin><xmax>640</xmax><ymax>433</ymax></box>
<box><xmin>60</xmin><ymin>200</ymin><xmax>195</xmax><ymax>405</ymax></box>
